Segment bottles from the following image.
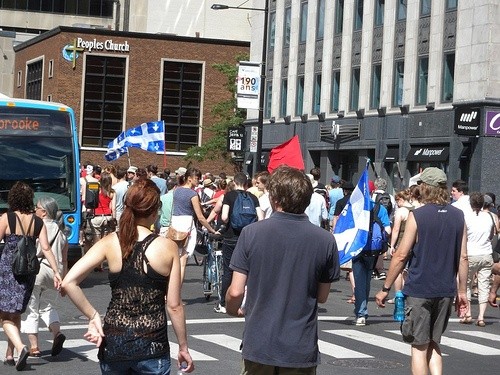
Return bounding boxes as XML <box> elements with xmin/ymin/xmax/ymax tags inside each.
<box><xmin>176</xmin><ymin>366</ymin><xmax>187</xmax><ymax>375</ymax></box>
<box><xmin>392</xmin><ymin>290</ymin><xmax>406</xmax><ymax>321</ymax></box>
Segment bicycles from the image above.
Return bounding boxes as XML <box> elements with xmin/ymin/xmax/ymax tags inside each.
<box><xmin>193</xmin><ymin>205</ymin><xmax>225</xmax><ymax>300</ymax></box>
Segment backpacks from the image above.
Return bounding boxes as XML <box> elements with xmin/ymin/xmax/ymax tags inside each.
<box><xmin>230</xmin><ymin>190</ymin><xmax>259</xmax><ymax>233</ymax></box>
<box><xmin>11</xmin><ymin>212</ymin><xmax>40</xmax><ymax>285</ymax></box>
<box><xmin>356</xmin><ymin>192</ymin><xmax>393</xmax><ymax>257</ymax></box>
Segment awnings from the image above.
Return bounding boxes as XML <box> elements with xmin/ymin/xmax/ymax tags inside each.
<box><xmin>383</xmin><ymin>147</ymin><xmax>399</xmax><ymax>161</ymax></box>
<box><xmin>404</xmin><ymin>146</ymin><xmax>448</xmax><ymax>162</ymax></box>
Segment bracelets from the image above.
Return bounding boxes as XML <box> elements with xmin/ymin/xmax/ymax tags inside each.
<box><xmin>382</xmin><ymin>285</ymin><xmax>391</xmax><ymax>292</ymax></box>
<box><xmin>92</xmin><ymin>311</ymin><xmax>98</xmax><ymax>319</ymax></box>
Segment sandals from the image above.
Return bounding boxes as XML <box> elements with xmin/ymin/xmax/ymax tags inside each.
<box><xmin>30</xmin><ymin>331</ymin><xmax>66</xmax><ymax>356</ymax></box>
<box><xmin>347</xmin><ymin>298</ymin><xmax>356</xmax><ymax>303</ymax></box>
<box><xmin>3</xmin><ymin>345</ymin><xmax>30</xmax><ymax>371</ymax></box>
<box><xmin>459</xmin><ymin>316</ymin><xmax>485</xmax><ymax>326</ymax></box>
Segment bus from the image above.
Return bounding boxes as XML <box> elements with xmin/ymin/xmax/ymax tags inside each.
<box><xmin>0</xmin><ymin>94</ymin><xmax>84</xmax><ymax>268</ymax></box>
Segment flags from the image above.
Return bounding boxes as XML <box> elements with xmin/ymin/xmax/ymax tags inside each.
<box><xmin>268</xmin><ymin>134</ymin><xmax>305</xmax><ymax>174</ymax></box>
<box><xmin>331</xmin><ymin>160</ymin><xmax>371</xmax><ymax>264</ymax></box>
<box><xmin>105</xmin><ymin>120</ymin><xmax>164</xmax><ymax>161</ymax></box>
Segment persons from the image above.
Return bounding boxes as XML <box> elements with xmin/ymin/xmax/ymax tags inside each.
<box><xmin>0</xmin><ymin>163</ymin><xmax>500</xmax><ymax>375</ymax></box>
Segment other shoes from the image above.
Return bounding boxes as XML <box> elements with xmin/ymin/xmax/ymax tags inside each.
<box><xmin>382</xmin><ymin>256</ymin><xmax>388</xmax><ymax>260</ymax></box>
<box><xmin>352</xmin><ymin>316</ymin><xmax>366</xmax><ymax>326</ymax></box>
<box><xmin>214</xmin><ymin>304</ymin><xmax>227</xmax><ymax>314</ymax></box>
<box><xmin>371</xmin><ymin>271</ymin><xmax>387</xmax><ymax>280</ymax></box>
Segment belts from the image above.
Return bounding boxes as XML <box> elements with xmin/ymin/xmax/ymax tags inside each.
<box><xmin>95</xmin><ymin>213</ymin><xmax>112</xmax><ymax>216</ymax></box>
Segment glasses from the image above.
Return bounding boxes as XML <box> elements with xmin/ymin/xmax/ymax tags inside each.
<box><xmin>159</xmin><ymin>200</ymin><xmax>162</xmax><ymax>208</ymax></box>
<box><xmin>34</xmin><ymin>206</ymin><xmax>41</xmax><ymax>211</ymax></box>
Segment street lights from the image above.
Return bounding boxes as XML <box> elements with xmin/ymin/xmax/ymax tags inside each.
<box><xmin>209</xmin><ymin>4</ymin><xmax>270</xmax><ymax>176</ymax></box>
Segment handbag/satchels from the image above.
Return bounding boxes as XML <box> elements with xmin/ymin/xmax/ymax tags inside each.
<box><xmin>491</xmin><ymin>232</ymin><xmax>500</xmax><ymax>263</ymax></box>
<box><xmin>196</xmin><ymin>239</ymin><xmax>209</xmax><ymax>254</ymax></box>
<box><xmin>108</xmin><ymin>218</ymin><xmax>118</xmax><ymax>228</ymax></box>
<box><xmin>166</xmin><ymin>227</ymin><xmax>191</xmax><ymax>248</ymax></box>
<box><xmin>201</xmin><ymin>189</ymin><xmax>213</xmax><ymax>207</ymax></box>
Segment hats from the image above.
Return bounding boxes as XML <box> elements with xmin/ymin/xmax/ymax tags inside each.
<box><xmin>305</xmin><ymin>173</ymin><xmax>354</xmax><ymax>189</ymax></box>
<box><xmin>408</xmin><ymin>167</ymin><xmax>447</xmax><ymax>187</ymax></box>
<box><xmin>484</xmin><ymin>195</ymin><xmax>493</xmax><ymax>205</ymax></box>
<box><xmin>174</xmin><ymin>166</ymin><xmax>227</xmax><ymax>185</ymax></box>
<box><xmin>368</xmin><ymin>180</ymin><xmax>375</xmax><ymax>191</ymax></box>
<box><xmin>86</xmin><ymin>164</ymin><xmax>138</xmax><ymax>173</ymax></box>
